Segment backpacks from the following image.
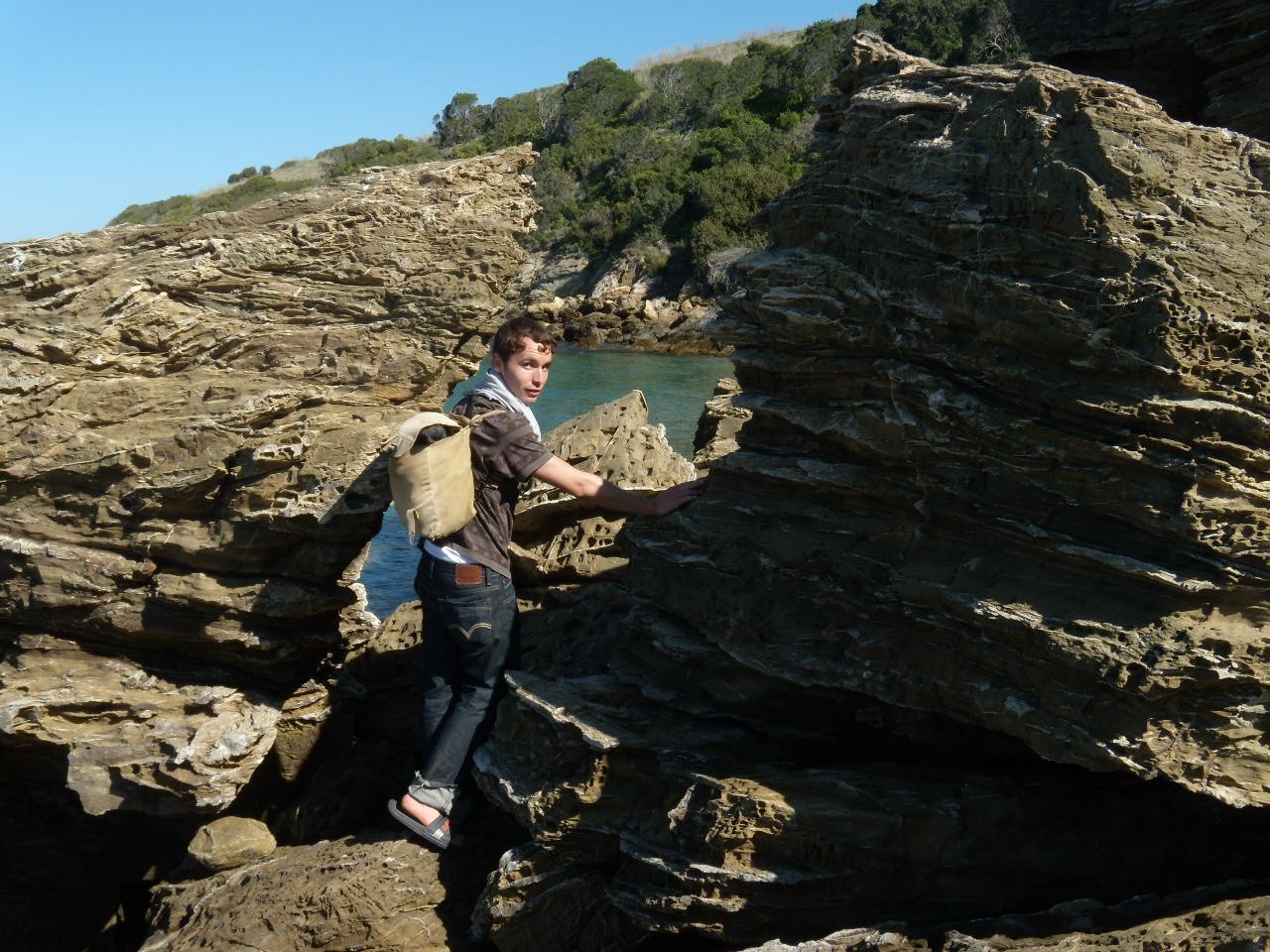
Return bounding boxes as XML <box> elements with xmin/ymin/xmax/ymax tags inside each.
<box><xmin>379</xmin><ymin>408</ymin><xmax>508</xmax><ymax>543</ymax></box>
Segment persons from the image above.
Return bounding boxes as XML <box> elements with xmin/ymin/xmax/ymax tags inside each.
<box><xmin>386</xmin><ymin>317</ymin><xmax>702</xmax><ymax>852</ymax></box>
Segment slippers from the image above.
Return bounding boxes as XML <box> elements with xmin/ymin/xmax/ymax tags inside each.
<box><xmin>388</xmin><ymin>798</ymin><xmax>467</xmax><ymax>852</ymax></box>
